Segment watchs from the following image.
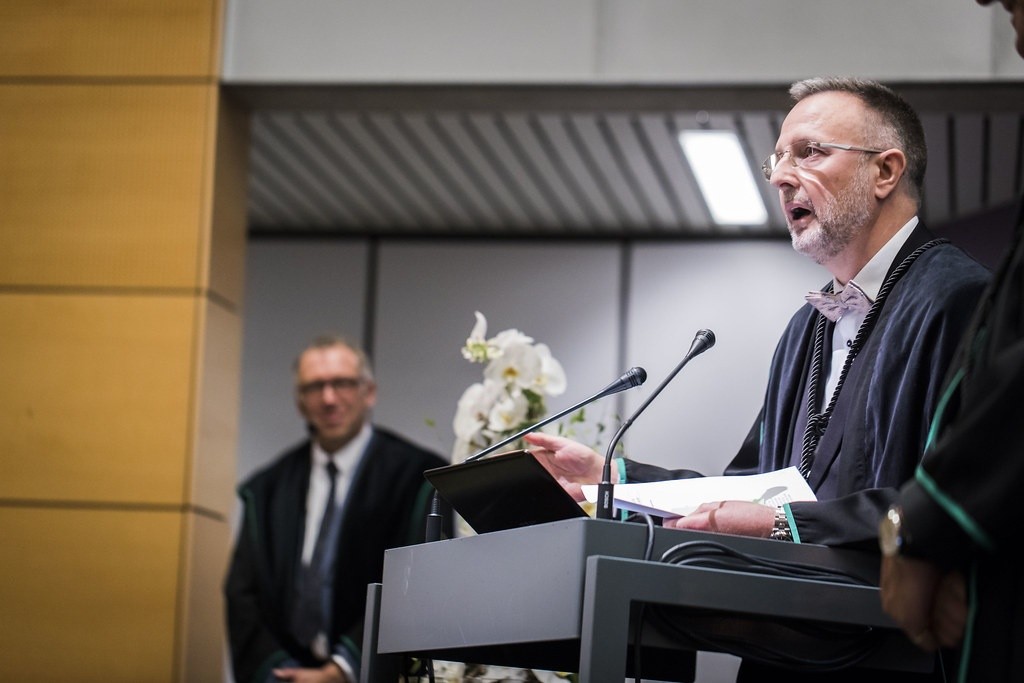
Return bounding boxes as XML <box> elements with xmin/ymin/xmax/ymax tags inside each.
<box><xmin>768</xmin><ymin>506</ymin><xmax>793</xmax><ymax>543</ymax></box>
<box><xmin>881</xmin><ymin>506</ymin><xmax>929</xmax><ymax>562</ymax></box>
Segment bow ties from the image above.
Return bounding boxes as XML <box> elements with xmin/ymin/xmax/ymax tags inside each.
<box><xmin>803</xmin><ymin>282</ymin><xmax>872</xmax><ymax>322</ymax></box>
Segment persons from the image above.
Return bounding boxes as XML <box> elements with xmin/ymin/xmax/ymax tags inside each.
<box><xmin>523</xmin><ymin>76</ymin><xmax>1024</xmax><ymax>683</ymax></box>
<box><xmin>218</xmin><ymin>336</ymin><xmax>455</xmax><ymax>683</ymax></box>
<box><xmin>880</xmin><ymin>0</ymin><xmax>1024</xmax><ymax>683</ymax></box>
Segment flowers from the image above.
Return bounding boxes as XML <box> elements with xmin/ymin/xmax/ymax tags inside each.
<box><xmin>448</xmin><ymin>310</ymin><xmax>624</xmax><ymax>536</ymax></box>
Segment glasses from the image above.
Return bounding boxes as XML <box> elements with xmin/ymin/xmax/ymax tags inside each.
<box><xmin>297</xmin><ymin>376</ymin><xmax>359</xmax><ymax>392</ymax></box>
<box><xmin>761</xmin><ymin>139</ymin><xmax>888</xmax><ymax>179</ymax></box>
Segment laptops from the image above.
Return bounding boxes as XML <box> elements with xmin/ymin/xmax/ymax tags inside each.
<box><xmin>423</xmin><ymin>449</ymin><xmax>591</xmax><ymax>534</ymax></box>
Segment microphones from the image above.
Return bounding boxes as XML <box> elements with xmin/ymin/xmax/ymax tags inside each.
<box><xmin>598</xmin><ymin>328</ymin><xmax>716</xmax><ymax>519</ymax></box>
<box><xmin>426</xmin><ymin>366</ymin><xmax>647</xmax><ymax>541</ymax></box>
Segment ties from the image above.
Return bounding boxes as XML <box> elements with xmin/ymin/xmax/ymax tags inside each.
<box><xmin>286</xmin><ymin>461</ymin><xmax>339</xmax><ymax>644</ymax></box>
